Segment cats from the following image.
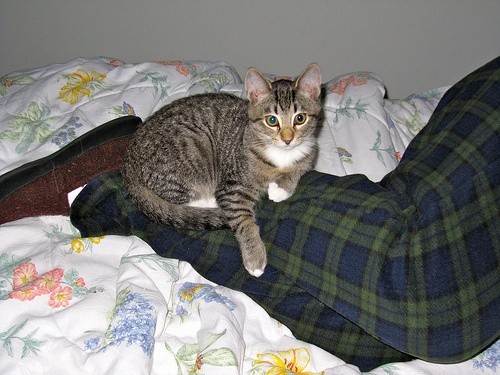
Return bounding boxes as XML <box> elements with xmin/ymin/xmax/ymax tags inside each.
<box><xmin>123</xmin><ymin>62</ymin><xmax>322</xmax><ymax>278</ymax></box>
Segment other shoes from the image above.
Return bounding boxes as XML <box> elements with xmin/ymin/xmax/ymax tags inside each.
<box><xmin>0</xmin><ymin>115</ymin><xmax>145</xmax><ymax>227</ymax></box>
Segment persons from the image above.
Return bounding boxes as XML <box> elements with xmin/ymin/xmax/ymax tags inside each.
<box><xmin>0</xmin><ymin>56</ymin><xmax>500</xmax><ymax>372</ymax></box>
